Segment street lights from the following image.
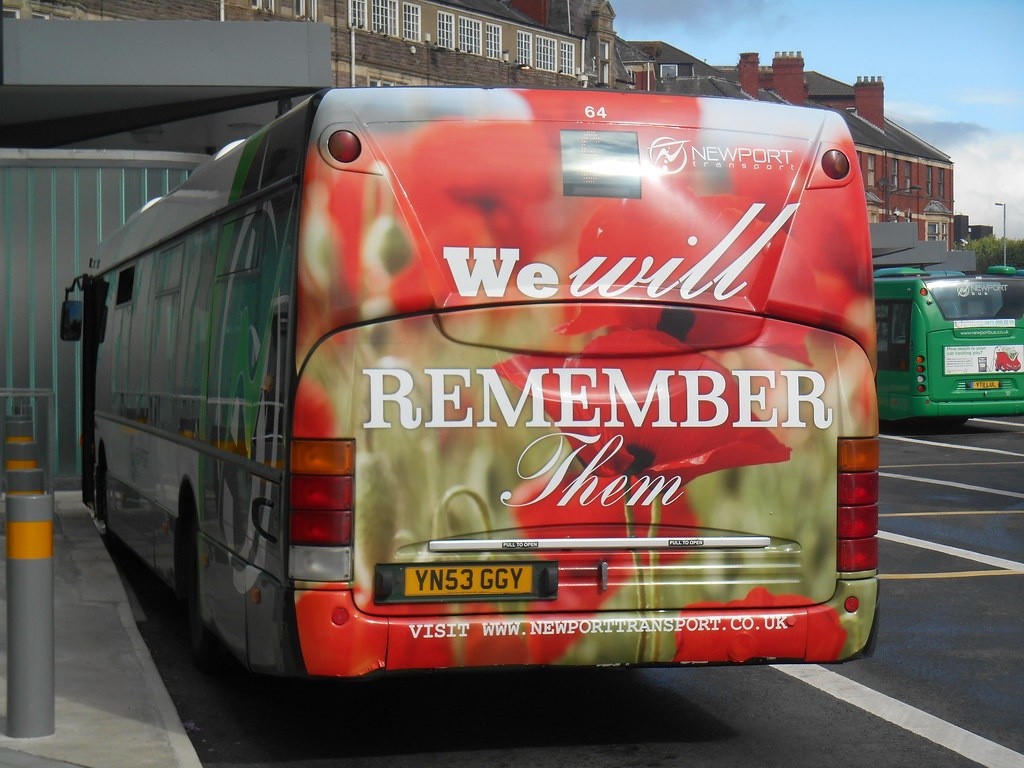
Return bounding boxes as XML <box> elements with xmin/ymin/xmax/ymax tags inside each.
<box><xmin>877</xmin><ymin>177</ymin><xmax>922</xmax><ymax>223</ymax></box>
<box><xmin>995</xmin><ymin>203</ymin><xmax>1007</xmax><ymax>267</ymax></box>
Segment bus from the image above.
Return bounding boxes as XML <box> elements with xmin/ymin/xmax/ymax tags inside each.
<box><xmin>873</xmin><ymin>266</ymin><xmax>1024</xmax><ymax>426</ymax></box>
<box><xmin>58</xmin><ymin>82</ymin><xmax>880</xmax><ymax>683</ymax></box>
